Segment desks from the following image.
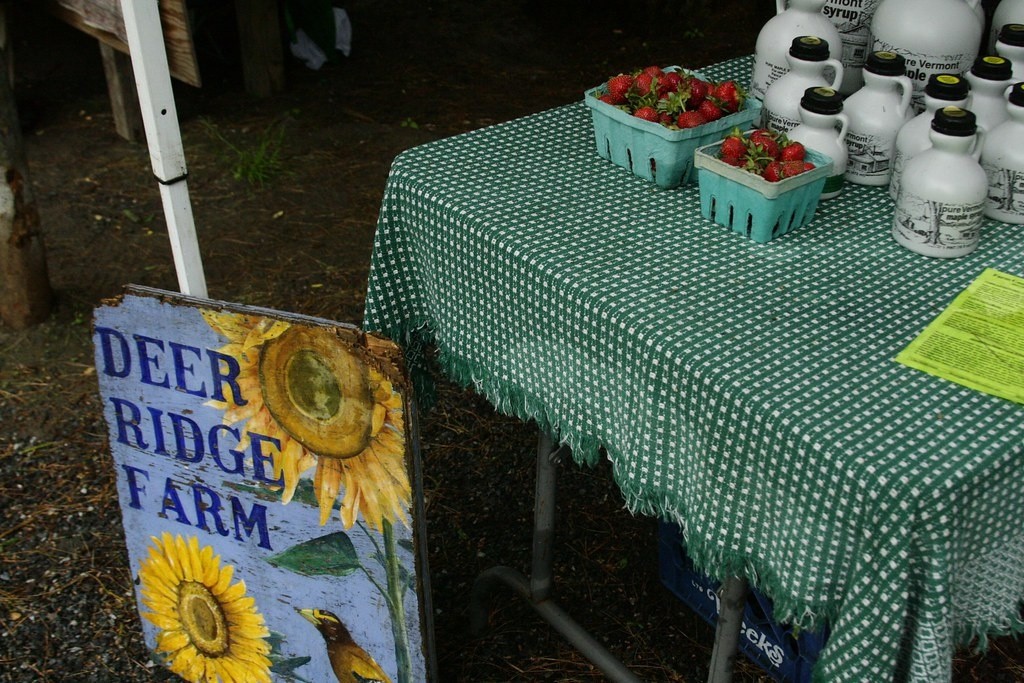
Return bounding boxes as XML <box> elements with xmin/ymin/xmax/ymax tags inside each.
<box><xmin>369</xmin><ymin>54</ymin><xmax>1024</xmax><ymax>681</ymax></box>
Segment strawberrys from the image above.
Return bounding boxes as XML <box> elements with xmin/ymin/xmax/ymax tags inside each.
<box><xmin>596</xmin><ymin>65</ymin><xmax>744</xmax><ymax>130</ymax></box>
<box><xmin>718</xmin><ymin>128</ymin><xmax>815</xmax><ymax>183</ymax></box>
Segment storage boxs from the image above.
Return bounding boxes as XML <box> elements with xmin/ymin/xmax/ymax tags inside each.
<box><xmin>694</xmin><ymin>128</ymin><xmax>833</xmax><ymax>241</ymax></box>
<box><xmin>584</xmin><ymin>66</ymin><xmax>760</xmax><ymax>190</ymax></box>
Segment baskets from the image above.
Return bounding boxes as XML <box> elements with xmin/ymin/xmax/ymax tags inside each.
<box><xmin>695</xmin><ymin>129</ymin><xmax>834</xmax><ymax>244</ymax></box>
<box><xmin>586</xmin><ymin>66</ymin><xmax>763</xmax><ymax>189</ymax></box>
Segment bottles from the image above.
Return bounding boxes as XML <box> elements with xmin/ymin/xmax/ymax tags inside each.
<box><xmin>750</xmin><ymin>0</ymin><xmax>1024</xmax><ymax>257</ymax></box>
